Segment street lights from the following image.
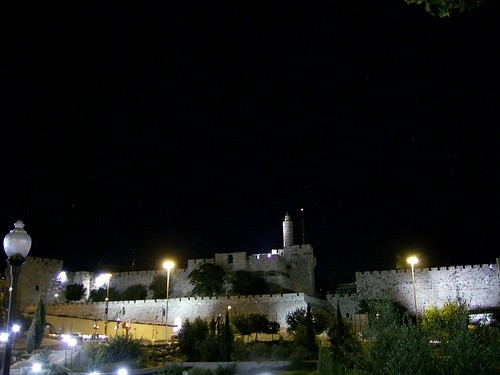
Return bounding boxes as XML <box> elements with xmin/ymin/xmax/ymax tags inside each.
<box><xmin>162</xmin><ymin>259</ymin><xmax>175</xmax><ymax>346</ymax></box>
<box><xmin>2</xmin><ymin>220</ymin><xmax>32</xmax><ymax>375</ymax></box>
<box><xmin>405</xmin><ymin>255</ymin><xmax>420</xmax><ymax>332</ymax></box>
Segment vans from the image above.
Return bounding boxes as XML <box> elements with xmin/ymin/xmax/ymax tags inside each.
<box><xmin>468</xmin><ymin>313</ymin><xmax>496</xmax><ymax>327</ymax></box>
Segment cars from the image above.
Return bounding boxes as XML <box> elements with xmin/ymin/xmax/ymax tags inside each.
<box><xmin>48</xmin><ymin>330</ymin><xmax>105</xmax><ymax>342</ymax></box>
